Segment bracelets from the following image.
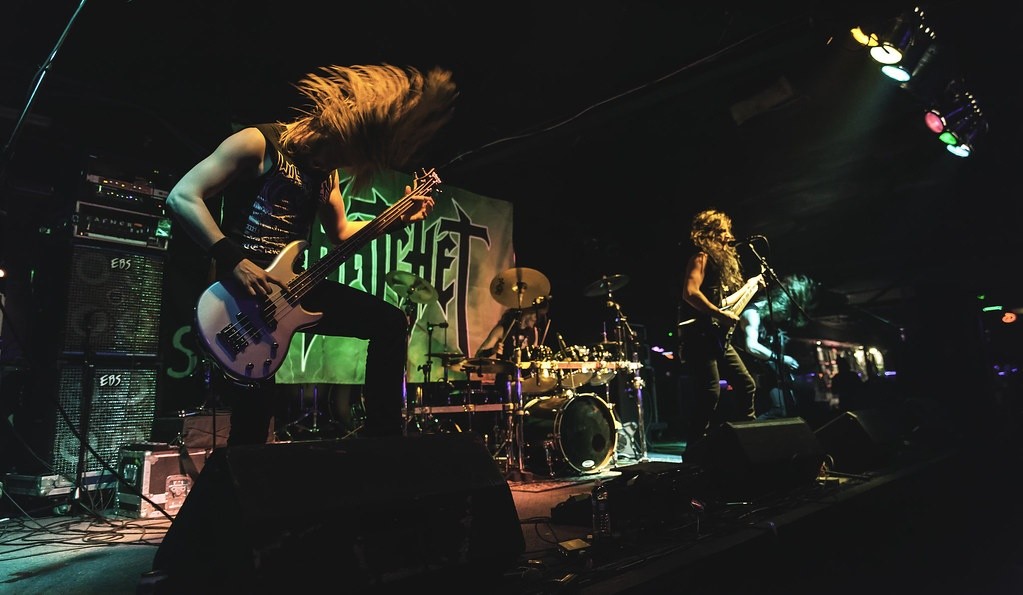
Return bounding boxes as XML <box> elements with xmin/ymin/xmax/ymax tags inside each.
<box><xmin>209</xmin><ymin>236</ymin><xmax>247</xmax><ymax>274</ymax></box>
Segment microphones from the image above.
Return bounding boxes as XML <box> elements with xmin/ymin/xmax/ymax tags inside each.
<box><xmin>728</xmin><ymin>234</ymin><xmax>762</xmax><ymax>248</ymax></box>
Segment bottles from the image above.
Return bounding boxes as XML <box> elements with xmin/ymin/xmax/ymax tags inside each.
<box><xmin>592</xmin><ymin>477</ymin><xmax>610</xmax><ymax>533</ymax></box>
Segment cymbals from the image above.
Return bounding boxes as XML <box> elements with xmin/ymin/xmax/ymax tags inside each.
<box><xmin>489</xmin><ymin>267</ymin><xmax>551</xmax><ymax>309</ymax></box>
<box><xmin>423</xmin><ymin>351</ymin><xmax>468</xmax><ymax>359</ymax></box>
<box><xmin>449</xmin><ymin>358</ymin><xmax>514</xmax><ymax>375</ymax></box>
<box><xmin>583</xmin><ymin>274</ymin><xmax>630</xmax><ymax>297</ymax></box>
<box><xmin>588</xmin><ymin>341</ymin><xmax>619</xmax><ymax>349</ymax></box>
<box><xmin>383</xmin><ymin>269</ymin><xmax>441</xmax><ymax>305</ymax></box>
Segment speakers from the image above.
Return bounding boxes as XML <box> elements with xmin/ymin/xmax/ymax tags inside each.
<box><xmin>813</xmin><ymin>407</ymin><xmax>898</xmax><ymax>467</ymax></box>
<box><xmin>683</xmin><ymin>417</ymin><xmax>826</xmax><ymax>495</ymax></box>
<box><xmin>0</xmin><ymin>240</ymin><xmax>169</xmax><ymax>358</ymax></box>
<box><xmin>153</xmin><ymin>432</ymin><xmax>525</xmax><ymax>594</ymax></box>
<box><xmin>0</xmin><ymin>359</ymin><xmax>162</xmax><ymax>496</ymax></box>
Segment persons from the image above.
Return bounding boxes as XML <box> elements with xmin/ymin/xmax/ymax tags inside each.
<box><xmin>164</xmin><ymin>63</ymin><xmax>461</xmax><ymax>446</ymax></box>
<box><xmin>475</xmin><ymin>307</ymin><xmax>542</xmax><ymax>358</ymax></box>
<box><xmin>737</xmin><ymin>274</ymin><xmax>817</xmax><ymax>423</ymax></box>
<box><xmin>673</xmin><ymin>209</ymin><xmax>758</xmax><ymax>454</ymax></box>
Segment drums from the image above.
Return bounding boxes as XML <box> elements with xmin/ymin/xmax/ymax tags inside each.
<box><xmin>553</xmin><ymin>345</ymin><xmax>599</xmax><ymax>389</ymax></box>
<box><xmin>585</xmin><ymin>344</ymin><xmax>619</xmax><ymax>386</ymax></box>
<box><xmin>509</xmin><ymin>345</ymin><xmax>561</xmax><ymax>396</ymax></box>
<box><xmin>514</xmin><ymin>390</ymin><xmax>620</xmax><ymax>479</ymax></box>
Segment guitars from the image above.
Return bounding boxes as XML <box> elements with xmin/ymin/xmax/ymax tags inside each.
<box><xmin>710</xmin><ymin>263</ymin><xmax>773</xmax><ymax>357</ymax></box>
<box><xmin>191</xmin><ymin>166</ymin><xmax>445</xmax><ymax>375</ymax></box>
